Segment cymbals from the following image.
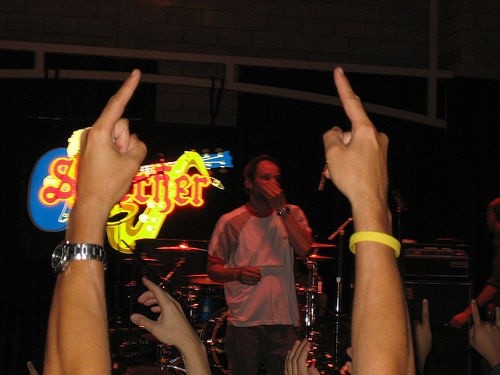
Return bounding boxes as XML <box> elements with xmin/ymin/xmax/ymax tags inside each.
<box><xmin>310</xmin><ymin>243</ymin><xmax>337</xmax><ymax>247</ymax></box>
<box><xmin>154</xmin><ymin>246</ymin><xmax>207</xmax><ymax>251</ymax></box>
<box><xmin>295</xmin><ymin>254</ymin><xmax>333</xmax><ymax>259</ymax></box>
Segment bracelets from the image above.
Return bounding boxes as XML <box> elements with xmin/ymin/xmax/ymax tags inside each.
<box><xmin>276</xmin><ymin>207</ymin><xmax>289</xmax><ymax>216</ymax></box>
<box><xmin>349</xmin><ymin>231</ymin><xmax>401</xmax><ymax>258</ymax></box>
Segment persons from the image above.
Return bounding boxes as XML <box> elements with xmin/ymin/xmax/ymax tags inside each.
<box><xmin>129</xmin><ymin>276</ymin><xmax>212</xmax><ymax>375</ymax></box>
<box><xmin>285</xmin><ymin>298</ymin><xmax>500</xmax><ymax>375</ymax></box>
<box><xmin>208</xmin><ymin>155</ymin><xmax>312</xmax><ymax>375</ymax></box>
<box><xmin>449</xmin><ymin>198</ymin><xmax>500</xmax><ymax>328</ymax></box>
<box><xmin>44</xmin><ymin>67</ymin><xmax>416</xmax><ymax>375</ymax></box>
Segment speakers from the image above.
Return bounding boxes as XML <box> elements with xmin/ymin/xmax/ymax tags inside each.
<box><xmin>403</xmin><ymin>280</ymin><xmax>473</xmax><ymax>375</ymax></box>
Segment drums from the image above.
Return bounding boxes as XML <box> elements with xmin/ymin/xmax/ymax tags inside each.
<box><xmin>200</xmin><ymin>308</ymin><xmax>230</xmax><ymax>375</ymax></box>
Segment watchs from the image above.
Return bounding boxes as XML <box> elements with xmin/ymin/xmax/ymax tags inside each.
<box><xmin>51</xmin><ymin>240</ymin><xmax>107</xmax><ymax>272</ymax></box>
